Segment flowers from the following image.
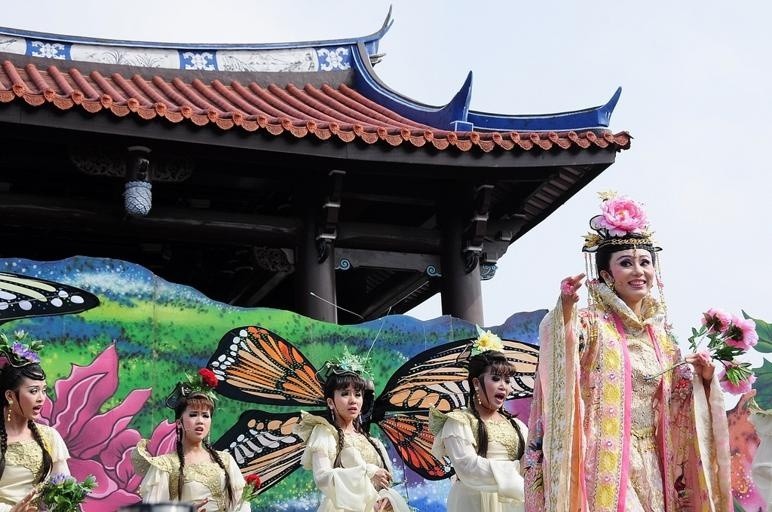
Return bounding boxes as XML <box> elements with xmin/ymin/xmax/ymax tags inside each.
<box><xmin>0</xmin><ymin>330</ymin><xmax>48</xmax><ymax>367</ymax></box>
<box><xmin>592</xmin><ymin>189</ymin><xmax>654</xmax><ymax>241</ymax></box>
<box><xmin>235</xmin><ymin>472</ymin><xmax>262</xmax><ymax>512</ymax></box>
<box><xmin>467</xmin><ymin>324</ymin><xmax>508</xmax><ymax>356</ymax></box>
<box><xmin>29</xmin><ymin>472</ymin><xmax>100</xmax><ymax>512</ymax></box>
<box><xmin>186</xmin><ymin>366</ymin><xmax>219</xmax><ymax>398</ymax></box>
<box><xmin>642</xmin><ymin>306</ymin><xmax>761</xmax><ymax>387</ymax></box>
<box><xmin>332</xmin><ymin>345</ymin><xmax>377</xmax><ymax>378</ymax></box>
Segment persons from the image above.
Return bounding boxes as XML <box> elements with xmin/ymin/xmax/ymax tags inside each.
<box><xmin>427</xmin><ymin>328</ymin><xmax>531</xmax><ymax>512</ymax></box>
<box><xmin>290</xmin><ymin>348</ymin><xmax>412</xmax><ymax>512</ymax></box>
<box><xmin>521</xmin><ymin>188</ymin><xmax>738</xmax><ymax>511</ymax></box>
<box><xmin>1</xmin><ymin>329</ymin><xmax>79</xmax><ymax>512</ymax></box>
<box><xmin>725</xmin><ymin>387</ymin><xmax>758</xmax><ymax>457</ymax></box>
<box><xmin>138</xmin><ymin>367</ymin><xmax>256</xmax><ymax>511</ymax></box>
